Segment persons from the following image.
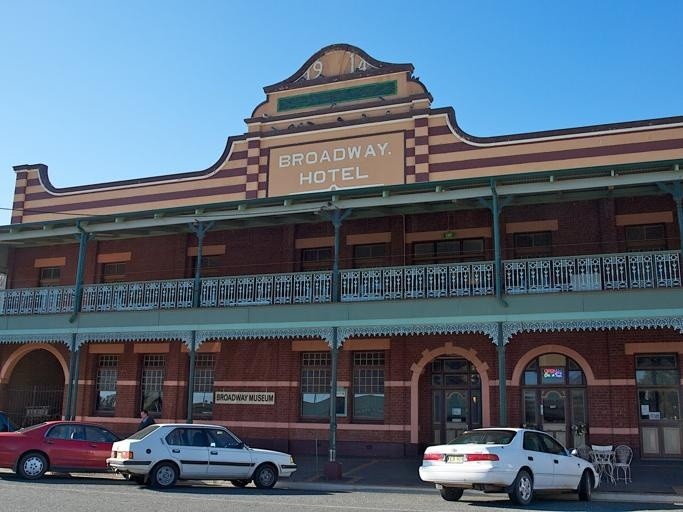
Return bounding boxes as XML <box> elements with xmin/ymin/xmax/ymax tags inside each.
<box><xmin>135</xmin><ymin>407</ymin><xmax>156</xmax><ymax>431</ymax></box>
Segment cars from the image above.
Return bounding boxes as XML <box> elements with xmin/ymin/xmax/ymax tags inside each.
<box><xmin>0</xmin><ymin>411</ymin><xmax>18</xmax><ymax>432</ymax></box>
<box><xmin>103</xmin><ymin>422</ymin><xmax>299</xmax><ymax>490</ymax></box>
<box><xmin>0</xmin><ymin>420</ymin><xmax>124</xmax><ymax>480</ymax></box>
<box><xmin>416</xmin><ymin>426</ymin><xmax>602</xmax><ymax>504</ymax></box>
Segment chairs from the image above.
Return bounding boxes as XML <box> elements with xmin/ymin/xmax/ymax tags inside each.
<box><xmin>577</xmin><ymin>443</ymin><xmax>634</xmax><ymax>488</ymax></box>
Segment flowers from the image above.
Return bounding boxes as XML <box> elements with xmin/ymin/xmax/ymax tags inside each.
<box><xmin>569</xmin><ymin>420</ymin><xmax>588</xmax><ymax>442</ymax></box>
